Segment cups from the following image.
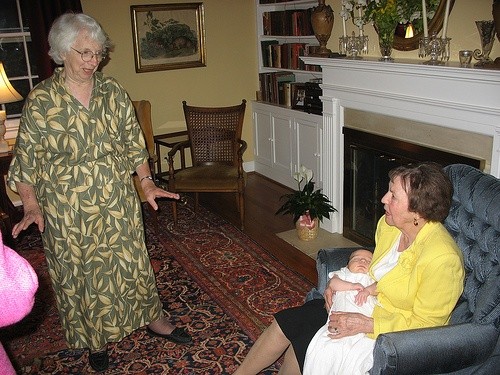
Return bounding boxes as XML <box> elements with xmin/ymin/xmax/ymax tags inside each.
<box><xmin>459</xmin><ymin>50</ymin><xmax>472</xmax><ymax>65</ymax></box>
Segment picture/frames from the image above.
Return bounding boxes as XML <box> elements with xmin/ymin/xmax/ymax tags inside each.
<box><xmin>130</xmin><ymin>2</ymin><xmax>206</xmax><ymax>73</ymax></box>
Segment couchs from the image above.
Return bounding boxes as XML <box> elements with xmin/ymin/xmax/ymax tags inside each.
<box><xmin>307</xmin><ymin>164</ymin><xmax>500</xmax><ymax>375</ymax></box>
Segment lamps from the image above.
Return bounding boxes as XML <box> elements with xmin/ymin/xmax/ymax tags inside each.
<box><xmin>0</xmin><ymin>62</ymin><xmax>24</xmax><ymax>153</ymax></box>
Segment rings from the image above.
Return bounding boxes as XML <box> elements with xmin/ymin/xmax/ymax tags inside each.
<box><xmin>335</xmin><ymin>327</ymin><xmax>339</xmax><ymax>334</ymax></box>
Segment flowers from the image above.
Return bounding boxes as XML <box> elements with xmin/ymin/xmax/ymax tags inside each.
<box><xmin>274</xmin><ymin>165</ymin><xmax>338</xmax><ymax>223</ymax></box>
<box><xmin>339</xmin><ymin>0</ymin><xmax>440</xmax><ymax>49</ymax></box>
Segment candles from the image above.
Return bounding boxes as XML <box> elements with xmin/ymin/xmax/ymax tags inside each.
<box><xmin>343</xmin><ymin>17</ymin><xmax>346</xmax><ymax>37</ymax></box>
<box><xmin>442</xmin><ymin>0</ymin><xmax>451</xmax><ymax>38</ymax></box>
<box><xmin>358</xmin><ymin>9</ymin><xmax>362</xmax><ymax>36</ymax></box>
<box><xmin>422</xmin><ymin>0</ymin><xmax>428</xmax><ymax>37</ymax></box>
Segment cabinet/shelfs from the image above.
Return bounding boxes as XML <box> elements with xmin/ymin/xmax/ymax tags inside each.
<box><xmin>251</xmin><ymin>0</ymin><xmax>322</xmax><ymax>196</ymax></box>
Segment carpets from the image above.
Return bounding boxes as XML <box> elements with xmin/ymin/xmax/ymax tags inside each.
<box><xmin>0</xmin><ymin>194</ymin><xmax>318</xmax><ymax>375</ymax></box>
<box><xmin>276</xmin><ymin>228</ymin><xmax>362</xmax><ymax>260</ymax></box>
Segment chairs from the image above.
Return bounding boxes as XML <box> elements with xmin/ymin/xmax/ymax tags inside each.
<box><xmin>167</xmin><ymin>99</ymin><xmax>248</xmax><ymax>231</ymax></box>
<box><xmin>132</xmin><ymin>100</ymin><xmax>159</xmax><ymax>238</ymax></box>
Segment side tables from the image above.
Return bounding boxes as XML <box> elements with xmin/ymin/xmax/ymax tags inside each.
<box><xmin>154</xmin><ymin>128</ymin><xmax>236</xmax><ymax>184</ymax></box>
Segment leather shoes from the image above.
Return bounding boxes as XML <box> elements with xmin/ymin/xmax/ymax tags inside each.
<box><xmin>146</xmin><ymin>324</ymin><xmax>192</xmax><ymax>345</ymax></box>
<box><xmin>89</xmin><ymin>345</ymin><xmax>109</xmax><ymax>372</ymax></box>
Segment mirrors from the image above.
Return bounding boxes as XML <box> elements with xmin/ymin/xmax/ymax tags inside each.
<box><xmin>373</xmin><ymin>0</ymin><xmax>455</xmax><ymax>51</ymax></box>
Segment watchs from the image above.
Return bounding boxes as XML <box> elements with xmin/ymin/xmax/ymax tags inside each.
<box><xmin>140</xmin><ymin>176</ymin><xmax>152</xmax><ymax>182</ymax></box>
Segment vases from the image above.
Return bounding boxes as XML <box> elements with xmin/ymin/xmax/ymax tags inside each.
<box><xmin>296</xmin><ymin>210</ymin><xmax>318</xmax><ymax>241</ymax></box>
<box><xmin>376</xmin><ymin>25</ymin><xmax>396</xmax><ymax>61</ymax></box>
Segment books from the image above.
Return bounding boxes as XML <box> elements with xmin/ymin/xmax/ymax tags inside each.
<box><xmin>261</xmin><ymin>9</ymin><xmax>322</xmax><ymax>116</ymax></box>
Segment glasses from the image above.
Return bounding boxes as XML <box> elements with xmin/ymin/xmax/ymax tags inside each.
<box><xmin>70</xmin><ymin>47</ymin><xmax>107</xmax><ymax>62</ymax></box>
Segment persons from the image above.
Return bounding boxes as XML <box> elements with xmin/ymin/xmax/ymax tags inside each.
<box><xmin>232</xmin><ymin>161</ymin><xmax>465</xmax><ymax>375</ymax></box>
<box><xmin>0</xmin><ymin>230</ymin><xmax>38</xmax><ymax>375</ymax></box>
<box><xmin>7</xmin><ymin>13</ymin><xmax>192</xmax><ymax>371</ymax></box>
